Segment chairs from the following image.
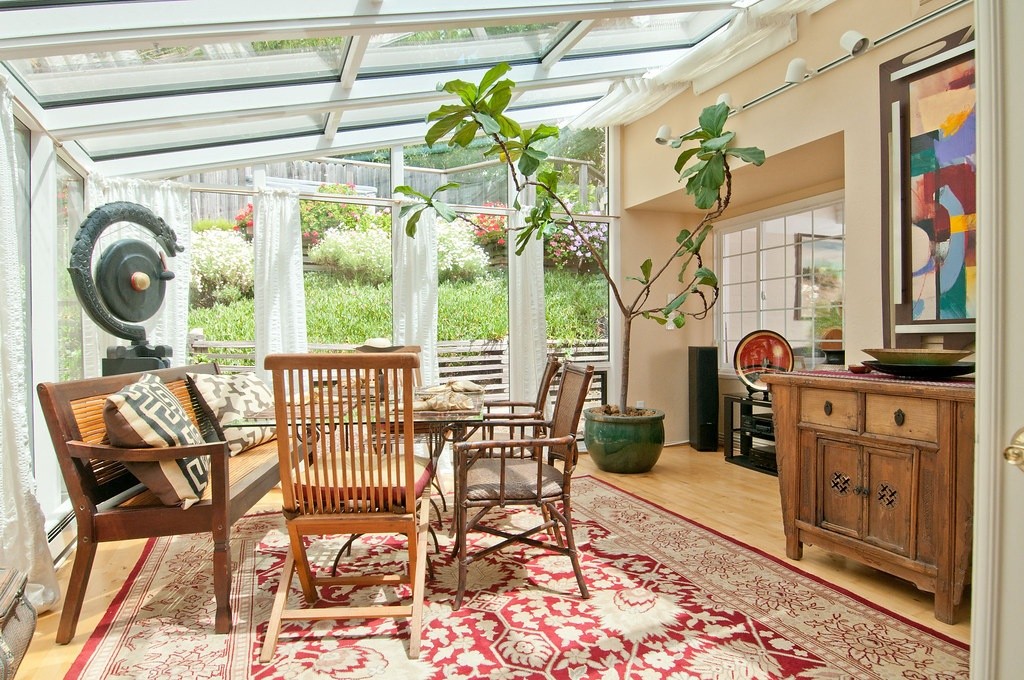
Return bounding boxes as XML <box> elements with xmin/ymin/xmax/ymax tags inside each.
<box><xmin>259</xmin><ymin>346</ymin><xmax>594</xmax><ymax>659</ymax></box>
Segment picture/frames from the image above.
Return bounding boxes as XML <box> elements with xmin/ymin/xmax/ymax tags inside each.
<box><xmin>794</xmin><ymin>233</ymin><xmax>844</xmax><ymax>321</ymax></box>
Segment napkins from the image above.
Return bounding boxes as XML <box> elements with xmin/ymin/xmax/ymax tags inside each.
<box><xmin>286</xmin><ymin>387</ymin><xmax>347</xmax><ymax>407</ymax></box>
<box><xmin>336</xmin><ymin>379</ymin><xmax>376</xmax><ymax>387</ymax></box>
<box><xmin>427</xmin><ymin>378</ymin><xmax>482</xmax><ymax>392</ymax></box>
<box><xmin>397</xmin><ymin>390</ymin><xmax>475</xmax><ymax>411</ymax></box>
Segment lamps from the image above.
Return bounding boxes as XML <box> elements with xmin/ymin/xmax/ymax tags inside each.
<box><xmin>785</xmin><ymin>57</ymin><xmax>819</xmax><ymax>84</ymax></box>
<box><xmin>655</xmin><ymin>125</ymin><xmax>676</xmax><ymax>146</ymax></box>
<box><xmin>840</xmin><ymin>30</ymin><xmax>874</xmax><ymax>58</ymax></box>
<box><xmin>715</xmin><ymin>93</ymin><xmax>744</xmax><ymax>114</ymax></box>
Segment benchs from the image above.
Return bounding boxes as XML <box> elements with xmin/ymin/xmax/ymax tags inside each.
<box><xmin>36</xmin><ymin>362</ymin><xmax>320</xmax><ymax>645</ymax></box>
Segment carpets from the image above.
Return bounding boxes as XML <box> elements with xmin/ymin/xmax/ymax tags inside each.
<box><xmin>61</xmin><ymin>473</ymin><xmax>971</xmax><ymax>680</ymax></box>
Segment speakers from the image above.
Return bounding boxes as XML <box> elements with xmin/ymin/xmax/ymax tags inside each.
<box><xmin>689</xmin><ymin>346</ymin><xmax>719</xmax><ymax>452</ymax></box>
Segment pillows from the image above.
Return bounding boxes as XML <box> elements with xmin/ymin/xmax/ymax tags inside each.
<box><xmin>185</xmin><ymin>372</ymin><xmax>295</xmax><ymax>457</ymax></box>
<box><xmin>103</xmin><ymin>373</ymin><xmax>210</xmax><ymax>512</ymax></box>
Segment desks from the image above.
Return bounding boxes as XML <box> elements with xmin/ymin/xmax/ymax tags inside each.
<box><xmin>555</xmin><ymin>372</ymin><xmax>608</xmax><ymax>406</ymax></box>
<box><xmin>224</xmin><ymin>384</ymin><xmax>487</xmax><ymax>580</ymax></box>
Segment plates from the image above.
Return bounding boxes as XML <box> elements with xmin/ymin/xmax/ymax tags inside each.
<box><xmin>861</xmin><ymin>347</ymin><xmax>975</xmax><ymax>365</ymax></box>
<box><xmin>860</xmin><ymin>360</ymin><xmax>974</xmax><ymax>378</ymax></box>
<box><xmin>735</xmin><ymin>330</ymin><xmax>795</xmax><ymax>390</ymax></box>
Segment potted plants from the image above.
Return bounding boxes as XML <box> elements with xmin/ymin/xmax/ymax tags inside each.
<box><xmin>391</xmin><ymin>63</ymin><xmax>765</xmax><ymax>475</ymax></box>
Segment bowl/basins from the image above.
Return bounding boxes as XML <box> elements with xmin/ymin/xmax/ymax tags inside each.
<box><xmin>850</xmin><ymin>367</ymin><xmax>870</xmax><ymax>374</ymax></box>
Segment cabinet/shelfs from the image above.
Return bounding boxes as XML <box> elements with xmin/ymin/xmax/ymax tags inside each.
<box><xmin>758</xmin><ymin>373</ymin><xmax>975</xmax><ymax>626</ymax></box>
<box><xmin>722</xmin><ymin>393</ymin><xmax>778</xmax><ymax>477</ymax></box>
